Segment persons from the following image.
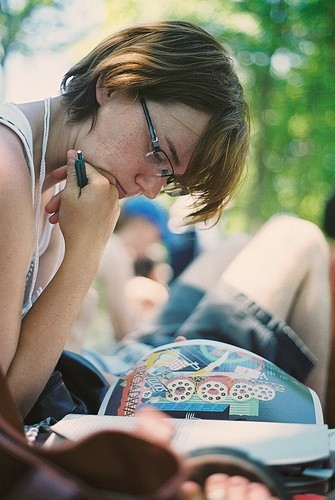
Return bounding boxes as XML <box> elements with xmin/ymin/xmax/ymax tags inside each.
<box><xmin>115</xmin><ymin>196</ymin><xmax>200</xmax><ymax>286</ymax></box>
<box><xmin>0</xmin><ymin>20</ymin><xmax>251</xmax><ymax>425</ymax></box>
<box><xmin>169</xmin><ymin>213</ymin><xmax>333</xmax><ymax>417</ymax></box>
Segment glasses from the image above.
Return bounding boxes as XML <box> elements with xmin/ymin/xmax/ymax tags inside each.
<box><xmin>128</xmin><ymin>90</ymin><xmax>183</xmax><ymax>196</ymax></box>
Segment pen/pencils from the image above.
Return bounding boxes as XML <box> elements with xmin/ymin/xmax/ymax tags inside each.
<box><xmin>74</xmin><ymin>150</ymin><xmax>87</xmax><ymax>188</ymax></box>
